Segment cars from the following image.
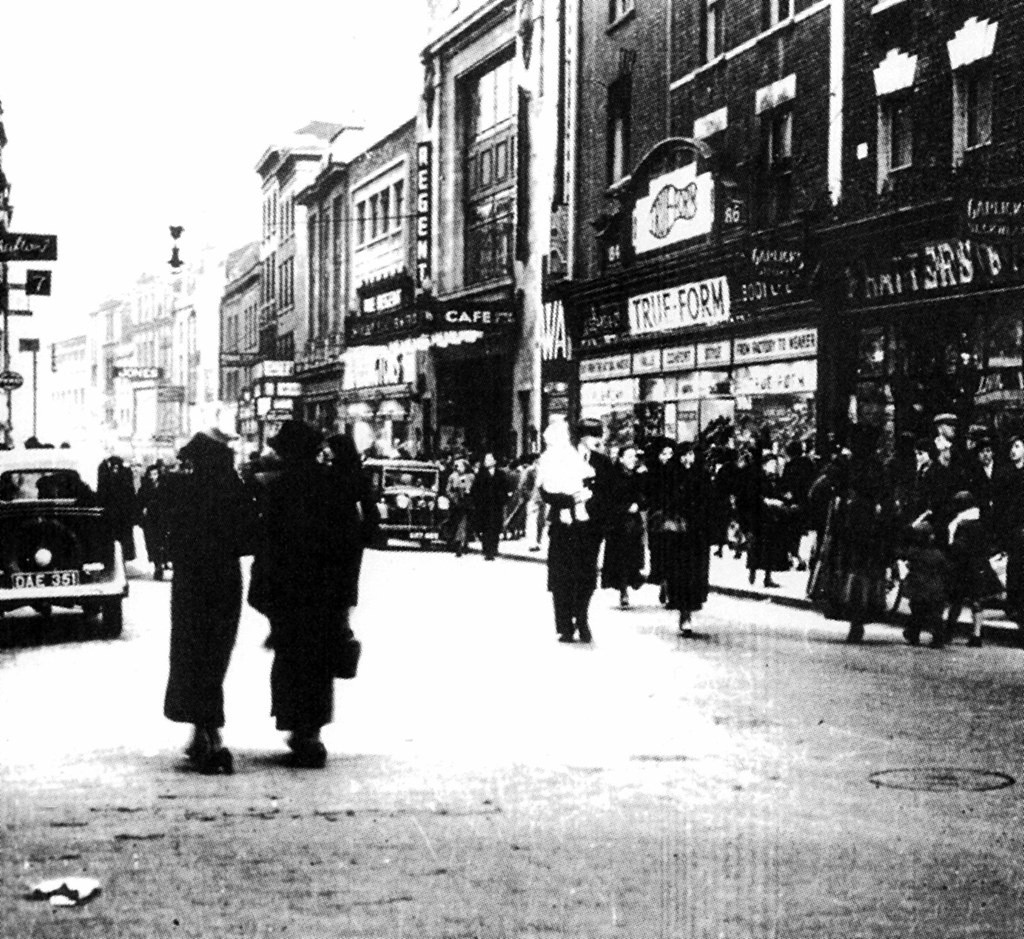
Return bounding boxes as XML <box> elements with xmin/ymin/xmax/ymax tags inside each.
<box><xmin>363</xmin><ymin>456</ymin><xmax>452</xmax><ymax>553</ymax></box>
<box><xmin>0</xmin><ymin>449</ymin><xmax>130</xmax><ymax>641</ymax></box>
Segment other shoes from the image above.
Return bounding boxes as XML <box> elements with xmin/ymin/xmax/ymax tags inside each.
<box><xmin>848</xmin><ymin>627</ymin><xmax>863</xmax><ymax>643</ymax></box>
<box><xmin>963</xmin><ymin>635</ymin><xmax>982</xmax><ymax>647</ymax></box>
<box><xmin>929</xmin><ymin>639</ymin><xmax>945</xmax><ymax>649</ymax></box>
<box><xmin>575</xmin><ymin>620</ymin><xmax>592</xmax><ymax>643</ymax></box>
<box><xmin>659</xmin><ymin>584</ymin><xmax>667</xmax><ymax>603</ymax></box>
<box><xmin>281</xmin><ymin>737</ymin><xmax>328</xmax><ymax>770</ymax></box>
<box><xmin>620</xmin><ymin>589</ymin><xmax>629</xmax><ymax>605</ymax></box>
<box><xmin>155</xmin><ymin>570</ymin><xmax>163</xmax><ymax>579</ymax></box>
<box><xmin>903</xmin><ymin>630</ymin><xmax>920</xmax><ymax>645</ymax></box>
<box><xmin>764</xmin><ymin>579</ymin><xmax>780</xmax><ymax>587</ymax></box>
<box><xmin>796</xmin><ymin>563</ymin><xmax>807</xmax><ymax>571</ymax></box>
<box><xmin>560</xmin><ymin>633</ymin><xmax>574</xmax><ymax>642</ymax></box>
<box><xmin>678</xmin><ymin>614</ymin><xmax>693</xmax><ymax>630</ymax></box>
<box><xmin>749</xmin><ymin>569</ymin><xmax>756</xmax><ymax>584</ymax></box>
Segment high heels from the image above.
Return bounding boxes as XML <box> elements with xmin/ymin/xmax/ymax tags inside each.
<box><xmin>185</xmin><ymin>743</ymin><xmax>235</xmax><ymax>775</ymax></box>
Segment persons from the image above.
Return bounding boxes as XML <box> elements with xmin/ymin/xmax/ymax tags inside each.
<box><xmin>534</xmin><ymin>412</ymin><xmax>598</xmax><ymax>526</ymax></box>
<box><xmin>437</xmin><ymin>412</ymin><xmax>1024</xmax><ymax>649</ymax></box>
<box><xmin>539</xmin><ymin>417</ymin><xmax>615</xmax><ymax>644</ymax></box>
<box><xmin>97</xmin><ymin>418</ymin><xmax>369</xmax><ymax>777</ymax></box>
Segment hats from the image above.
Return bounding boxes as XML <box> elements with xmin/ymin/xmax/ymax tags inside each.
<box><xmin>932</xmin><ymin>413</ymin><xmax>958</xmax><ymax>424</ymax></box>
<box><xmin>264</xmin><ymin>419</ymin><xmax>326</xmax><ymax>455</ymax></box>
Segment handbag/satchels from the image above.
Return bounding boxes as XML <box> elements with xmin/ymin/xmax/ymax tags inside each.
<box><xmin>334</xmin><ymin>613</ymin><xmax>361</xmax><ymax>678</ymax></box>
